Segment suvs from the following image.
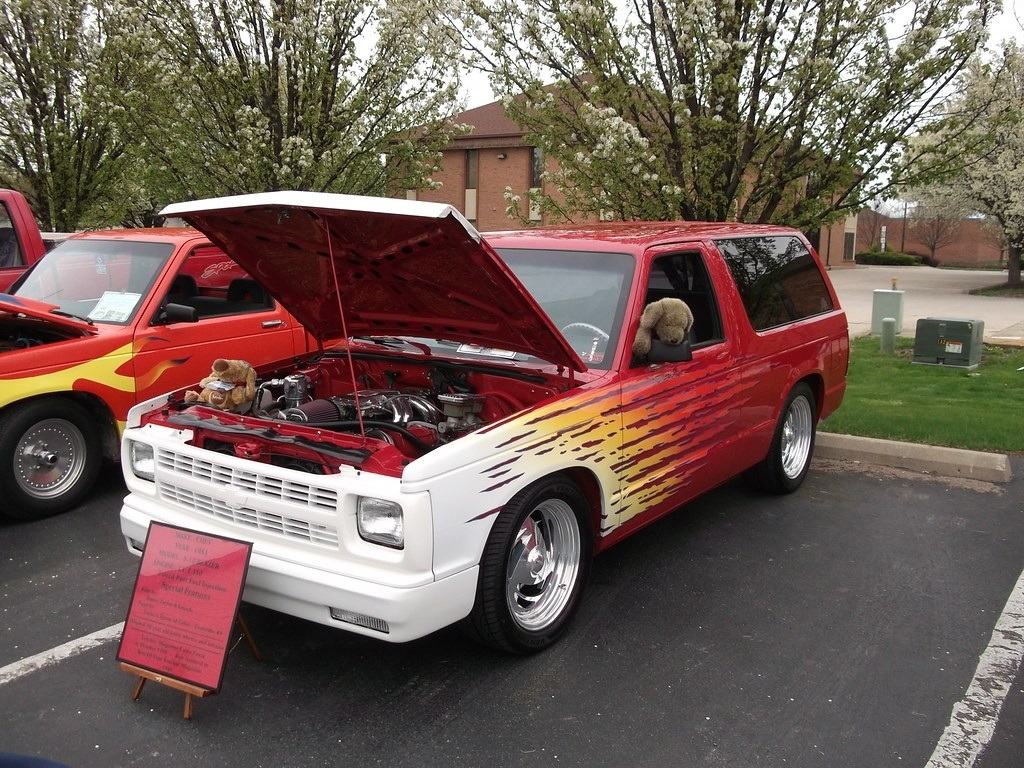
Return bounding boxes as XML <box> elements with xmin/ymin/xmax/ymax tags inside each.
<box><xmin>116</xmin><ymin>191</ymin><xmax>851</xmax><ymax>658</ymax></box>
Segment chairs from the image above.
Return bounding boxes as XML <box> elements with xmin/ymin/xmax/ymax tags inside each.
<box><xmin>227</xmin><ymin>277</ymin><xmax>274</xmax><ymax>312</ymax></box>
<box><xmin>165</xmin><ymin>273</ymin><xmax>196</xmax><ymax>303</ymax></box>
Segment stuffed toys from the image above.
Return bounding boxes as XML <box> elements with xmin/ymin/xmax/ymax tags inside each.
<box><xmin>184</xmin><ymin>358</ymin><xmax>257</xmax><ymax>410</ymax></box>
<box><xmin>632</xmin><ymin>297</ymin><xmax>693</xmax><ymax>356</ymax></box>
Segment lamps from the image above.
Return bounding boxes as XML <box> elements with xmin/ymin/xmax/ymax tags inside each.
<box><xmin>497</xmin><ymin>152</ymin><xmax>506</xmax><ymax>160</ymax></box>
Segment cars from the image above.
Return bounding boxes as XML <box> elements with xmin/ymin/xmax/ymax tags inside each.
<box><xmin>0</xmin><ymin>187</ymin><xmax>341</xmax><ymax>523</ymax></box>
